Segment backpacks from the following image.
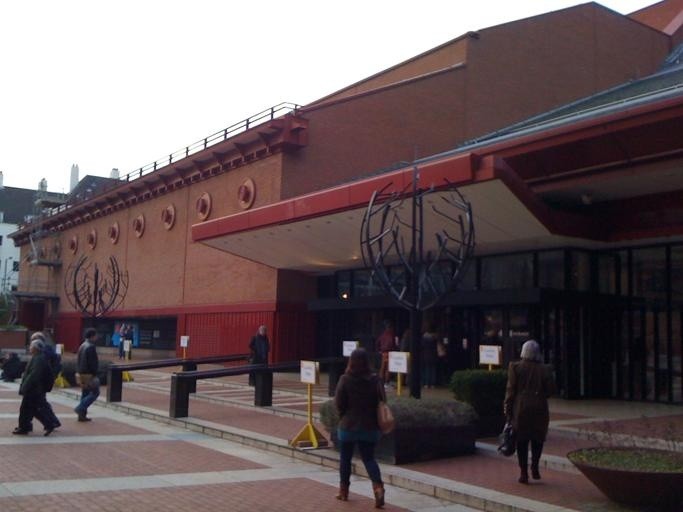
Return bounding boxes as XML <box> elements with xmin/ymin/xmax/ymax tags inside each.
<box><xmin>52</xmin><ymin>351</ymin><xmax>63</xmax><ymax>382</ymax></box>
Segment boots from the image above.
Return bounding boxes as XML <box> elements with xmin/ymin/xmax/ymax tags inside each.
<box><xmin>370</xmin><ymin>483</ymin><xmax>386</xmax><ymax>508</ymax></box>
<box><xmin>333</xmin><ymin>479</ymin><xmax>351</xmax><ymax>501</ymax></box>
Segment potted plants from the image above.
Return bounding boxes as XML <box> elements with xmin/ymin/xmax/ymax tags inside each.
<box><xmin>564</xmin><ymin>411</ymin><xmax>683</xmax><ymax>512</ymax></box>
<box><xmin>317</xmin><ymin>394</ymin><xmax>487</xmax><ymax>466</ymax></box>
<box><xmin>448</xmin><ymin>366</ymin><xmax>508</xmax><ymax>439</ymax></box>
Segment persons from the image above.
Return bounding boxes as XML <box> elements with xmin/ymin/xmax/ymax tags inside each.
<box><xmin>13</xmin><ymin>332</ymin><xmax>61</xmax><ymax>431</ymax></box>
<box><xmin>11</xmin><ymin>339</ymin><xmax>58</xmax><ymax>437</ymax></box>
<box><xmin>72</xmin><ymin>327</ymin><xmax>101</xmax><ymax>423</ymax></box>
<box><xmin>117</xmin><ymin>321</ymin><xmax>134</xmax><ymax>361</ymax></box>
<box><xmin>361</xmin><ymin>317</ymin><xmax>483</xmax><ymax>392</ymax></box>
<box><xmin>0</xmin><ymin>352</ymin><xmax>23</xmax><ymax>382</ymax></box>
<box><xmin>502</xmin><ymin>339</ymin><xmax>556</xmax><ymax>484</ymax></box>
<box><xmin>332</xmin><ymin>347</ymin><xmax>387</xmax><ymax>509</ymax></box>
<box><xmin>0</xmin><ymin>357</ymin><xmax>9</xmax><ymax>380</ymax></box>
<box><xmin>248</xmin><ymin>325</ymin><xmax>271</xmax><ymax>387</ymax></box>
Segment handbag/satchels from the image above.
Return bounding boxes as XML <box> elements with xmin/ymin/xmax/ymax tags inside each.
<box><xmin>72</xmin><ymin>371</ymin><xmax>101</xmax><ymax>390</ymax></box>
<box><xmin>494</xmin><ymin>430</ymin><xmax>516</xmax><ymax>457</ymax></box>
<box><xmin>373</xmin><ymin>399</ymin><xmax>396</xmax><ymax>435</ymax></box>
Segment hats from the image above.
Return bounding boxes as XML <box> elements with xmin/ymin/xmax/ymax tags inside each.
<box><xmin>30</xmin><ymin>340</ymin><xmax>45</xmax><ymax>351</ymax></box>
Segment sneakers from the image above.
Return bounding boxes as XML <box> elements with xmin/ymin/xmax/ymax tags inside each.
<box><xmin>11</xmin><ymin>422</ymin><xmax>34</xmax><ymax>435</ymax></box>
<box><xmin>518</xmin><ymin>466</ymin><xmax>529</xmax><ymax>484</ymax></box>
<box><xmin>41</xmin><ymin>420</ymin><xmax>61</xmax><ymax>438</ymax></box>
<box><xmin>530</xmin><ymin>461</ymin><xmax>541</xmax><ymax>481</ymax></box>
<box><xmin>71</xmin><ymin>408</ymin><xmax>90</xmax><ymax>422</ymax></box>
